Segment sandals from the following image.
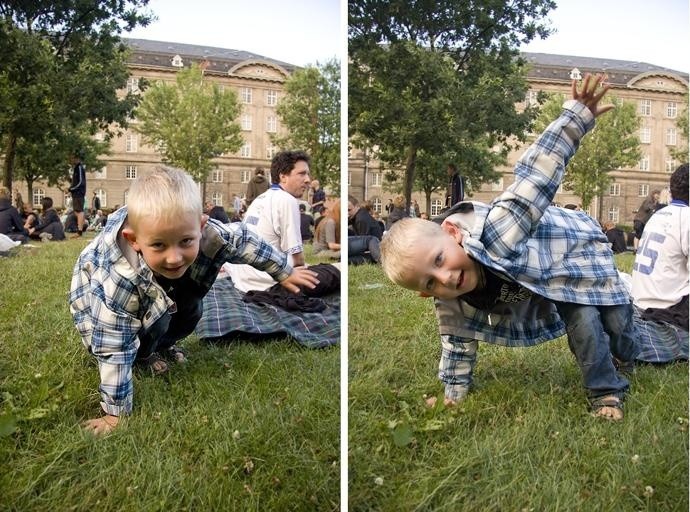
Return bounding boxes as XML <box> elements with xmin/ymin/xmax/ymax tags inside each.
<box><xmin>611</xmin><ymin>356</ymin><xmax>635</xmax><ymax>373</ymax></box>
<box><xmin>161</xmin><ymin>343</ymin><xmax>189</xmax><ymax>364</ymax></box>
<box><xmin>588</xmin><ymin>394</ymin><xmax>625</xmax><ymax>422</ymax></box>
<box><xmin>138</xmin><ymin>351</ymin><xmax>169</xmax><ymax>376</ymax></box>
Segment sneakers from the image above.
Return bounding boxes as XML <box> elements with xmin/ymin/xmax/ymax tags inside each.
<box><xmin>41</xmin><ymin>237</ymin><xmax>50</xmax><ymax>244</ymax></box>
<box><xmin>38</xmin><ymin>232</ymin><xmax>54</xmax><ymax>239</ymax></box>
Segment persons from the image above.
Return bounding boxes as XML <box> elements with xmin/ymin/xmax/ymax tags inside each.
<box><xmin>223</xmin><ymin>152</ymin><xmax>339</xmax><ymax>310</ymax></box>
<box><xmin>1</xmin><ymin>148</ymin><xmax>339</xmax><ymax>260</ymax></box>
<box><xmin>67</xmin><ymin>164</ymin><xmax>320</xmax><ymax>432</ymax></box>
<box><xmin>631</xmin><ymin>162</ymin><xmax>690</xmax><ymax>333</ymax></box>
<box><xmin>378</xmin><ymin>70</ymin><xmax>646</xmax><ymax>424</ymax></box>
<box><xmin>347</xmin><ymin>165</ymin><xmax>673</xmax><ymax>269</ymax></box>
